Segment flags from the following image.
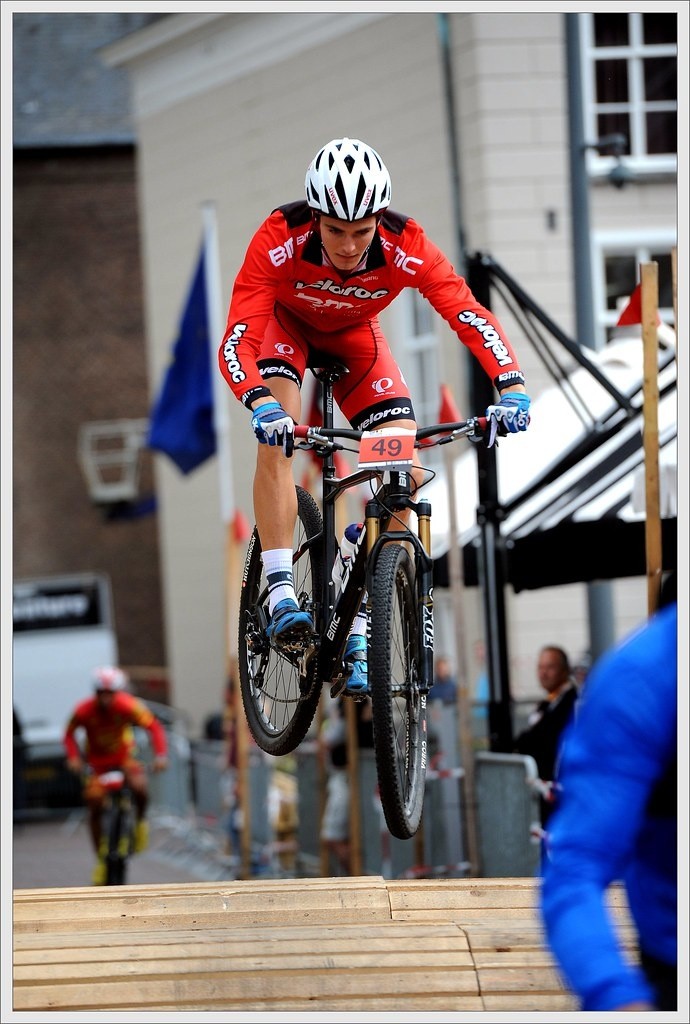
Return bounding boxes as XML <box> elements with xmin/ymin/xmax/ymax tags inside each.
<box><xmin>144</xmin><ymin>216</ymin><xmax>227</xmax><ymax>469</ymax></box>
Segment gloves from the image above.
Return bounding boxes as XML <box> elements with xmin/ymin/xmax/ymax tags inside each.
<box><xmin>252</xmin><ymin>398</ymin><xmax>298</xmax><ymax>457</ymax></box>
<box><xmin>483</xmin><ymin>393</ymin><xmax>528</xmax><ymax>447</ymax></box>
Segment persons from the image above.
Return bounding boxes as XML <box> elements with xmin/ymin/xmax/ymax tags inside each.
<box><xmin>428</xmin><ymin>655</ymin><xmax>457</xmax><ymax>705</ymax></box>
<box><xmin>514</xmin><ymin>647</ymin><xmax>578</xmax><ymax>832</ymax></box>
<box><xmin>318</xmin><ymin>694</ymin><xmax>376</xmax><ymax>878</ymax></box>
<box><xmin>216</xmin><ymin>136</ymin><xmax>530</xmax><ymax>696</ymax></box>
<box><xmin>540</xmin><ymin>575</ymin><xmax>677</xmax><ymax>1013</ymax></box>
<box><xmin>62</xmin><ymin>667</ymin><xmax>169</xmax><ymax>886</ymax></box>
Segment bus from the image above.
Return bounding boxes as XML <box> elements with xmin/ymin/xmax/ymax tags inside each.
<box><xmin>13</xmin><ymin>566</ymin><xmax>123</xmax><ymax>807</ymax></box>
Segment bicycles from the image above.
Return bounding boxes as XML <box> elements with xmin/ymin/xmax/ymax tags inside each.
<box><xmin>236</xmin><ymin>351</ymin><xmax>531</xmax><ymax>843</ymax></box>
<box><xmin>66</xmin><ymin>761</ymin><xmax>166</xmax><ymax>886</ymax></box>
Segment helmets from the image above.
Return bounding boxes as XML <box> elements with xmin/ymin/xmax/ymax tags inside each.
<box><xmin>94</xmin><ymin>665</ymin><xmax>127</xmax><ymax>692</ymax></box>
<box><xmin>305</xmin><ymin>137</ymin><xmax>391</xmax><ymax>215</ymax></box>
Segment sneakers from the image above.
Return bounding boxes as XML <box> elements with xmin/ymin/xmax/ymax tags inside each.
<box><xmin>265</xmin><ymin>599</ymin><xmax>312</xmax><ymax>646</ymax></box>
<box><xmin>345</xmin><ymin>633</ymin><xmax>370</xmax><ymax>696</ymax></box>
